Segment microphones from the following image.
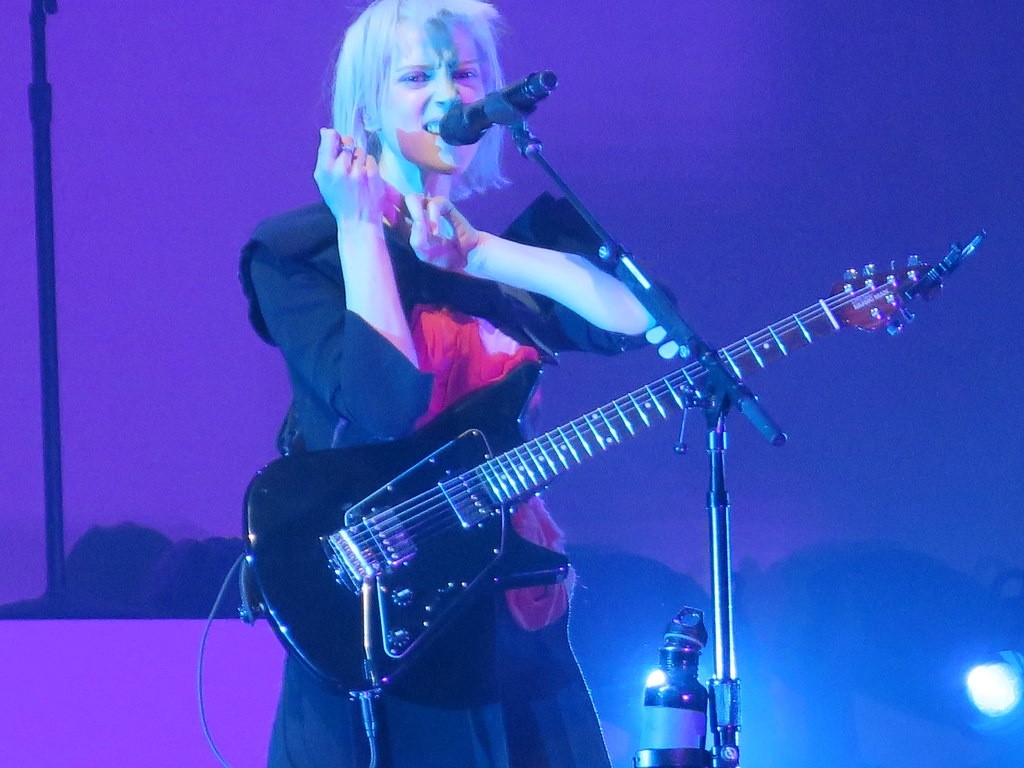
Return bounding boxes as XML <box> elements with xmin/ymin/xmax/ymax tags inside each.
<box><xmin>439</xmin><ymin>70</ymin><xmax>558</xmax><ymax>147</ymax></box>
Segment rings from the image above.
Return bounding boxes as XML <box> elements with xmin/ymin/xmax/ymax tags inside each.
<box><xmin>340</xmin><ymin>144</ymin><xmax>355</xmax><ymax>153</ymax></box>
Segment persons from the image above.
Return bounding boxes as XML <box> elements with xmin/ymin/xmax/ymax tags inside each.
<box><xmin>238</xmin><ymin>0</ymin><xmax>685</xmax><ymax>768</ymax></box>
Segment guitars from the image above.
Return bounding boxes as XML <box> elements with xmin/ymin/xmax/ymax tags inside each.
<box><xmin>236</xmin><ymin>224</ymin><xmax>992</xmax><ymax>705</ymax></box>
